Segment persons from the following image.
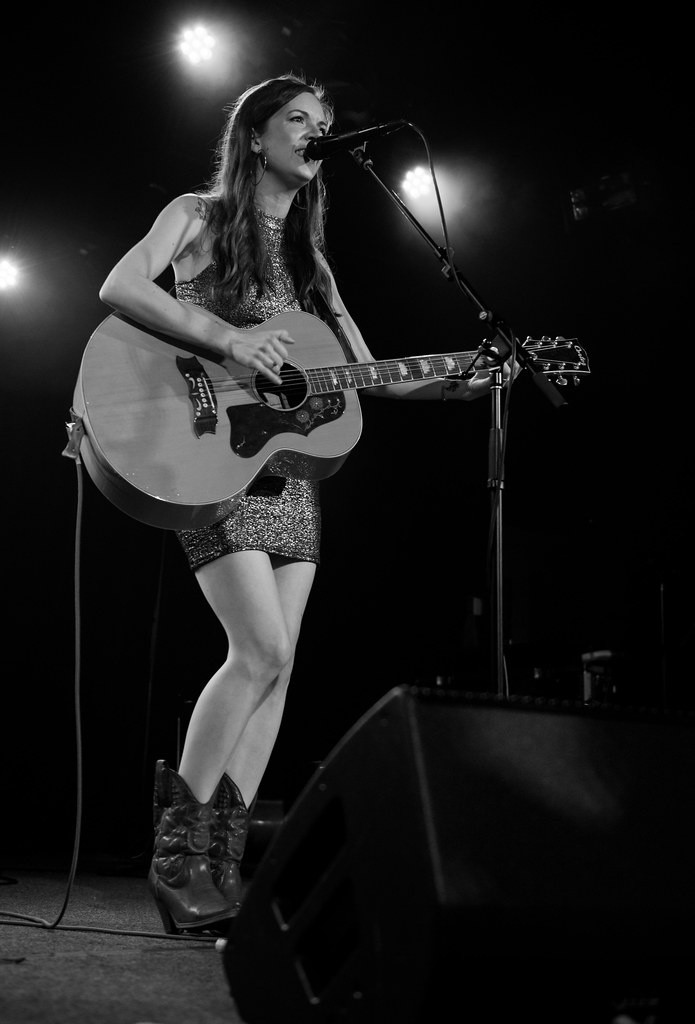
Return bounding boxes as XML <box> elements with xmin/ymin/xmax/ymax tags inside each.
<box><xmin>67</xmin><ymin>72</ymin><xmax>522</xmax><ymax>934</ymax></box>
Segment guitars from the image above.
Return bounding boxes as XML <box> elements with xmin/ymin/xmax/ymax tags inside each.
<box><xmin>67</xmin><ymin>300</ymin><xmax>595</xmax><ymax>533</ymax></box>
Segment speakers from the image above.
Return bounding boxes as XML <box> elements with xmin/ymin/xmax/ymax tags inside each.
<box><xmin>220</xmin><ymin>684</ymin><xmax>695</xmax><ymax>1024</ymax></box>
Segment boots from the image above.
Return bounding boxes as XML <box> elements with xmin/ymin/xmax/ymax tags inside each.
<box><xmin>209</xmin><ymin>772</ymin><xmax>249</xmax><ymax>909</ymax></box>
<box><xmin>147</xmin><ymin>759</ymin><xmax>239</xmax><ymax>935</ymax></box>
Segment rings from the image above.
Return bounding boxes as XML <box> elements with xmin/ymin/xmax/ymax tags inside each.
<box><xmin>271</xmin><ymin>361</ymin><xmax>277</xmax><ymax>370</ymax></box>
<box><xmin>502</xmin><ymin>378</ymin><xmax>510</xmax><ymax>383</ymax></box>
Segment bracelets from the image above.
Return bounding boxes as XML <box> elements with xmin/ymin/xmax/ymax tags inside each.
<box><xmin>441</xmin><ymin>377</ymin><xmax>448</xmax><ymax>402</ymax></box>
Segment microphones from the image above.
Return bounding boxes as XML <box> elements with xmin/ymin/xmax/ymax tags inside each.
<box><xmin>306</xmin><ymin>120</ymin><xmax>409</xmax><ymax>161</ymax></box>
